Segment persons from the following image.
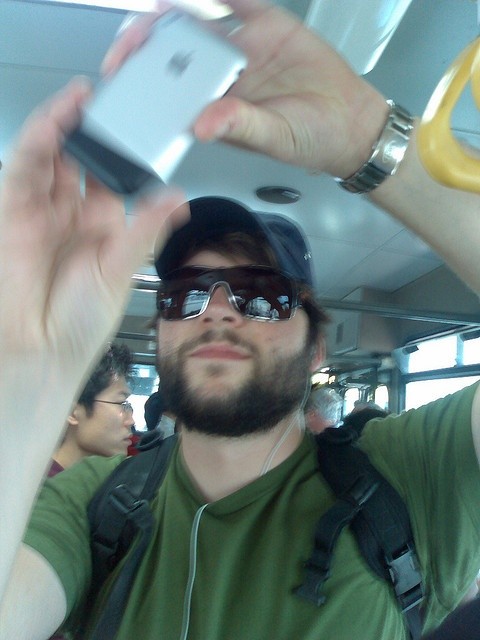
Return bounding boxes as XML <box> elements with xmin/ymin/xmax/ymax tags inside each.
<box><xmin>304</xmin><ymin>385</ymin><xmax>345</xmax><ymax>434</ymax></box>
<box><xmin>45</xmin><ymin>341</ymin><xmax>137</xmax><ymax>478</ymax></box>
<box><xmin>1</xmin><ymin>0</ymin><xmax>480</xmax><ymax>640</ymax></box>
<box><xmin>143</xmin><ymin>392</ymin><xmax>177</xmax><ymax>431</ymax></box>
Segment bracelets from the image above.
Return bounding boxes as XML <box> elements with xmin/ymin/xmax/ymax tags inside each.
<box><xmin>332</xmin><ymin>96</ymin><xmax>415</xmax><ymax>197</ymax></box>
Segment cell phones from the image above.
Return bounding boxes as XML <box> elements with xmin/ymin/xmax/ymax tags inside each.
<box><xmin>58</xmin><ymin>6</ymin><xmax>250</xmax><ymax>195</ymax></box>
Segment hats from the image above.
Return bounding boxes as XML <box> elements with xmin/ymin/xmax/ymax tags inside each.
<box><xmin>154</xmin><ymin>197</ymin><xmax>313</xmax><ymax>279</ymax></box>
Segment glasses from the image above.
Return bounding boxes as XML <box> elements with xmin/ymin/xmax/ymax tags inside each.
<box><xmin>92</xmin><ymin>399</ymin><xmax>133</xmax><ymax>410</ymax></box>
<box><xmin>157</xmin><ymin>265</ymin><xmax>297</xmax><ymax>321</ymax></box>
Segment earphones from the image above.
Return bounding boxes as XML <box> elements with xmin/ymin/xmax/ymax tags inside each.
<box><xmin>311</xmin><ymin>349</ymin><xmax>316</xmax><ymax>359</ymax></box>
